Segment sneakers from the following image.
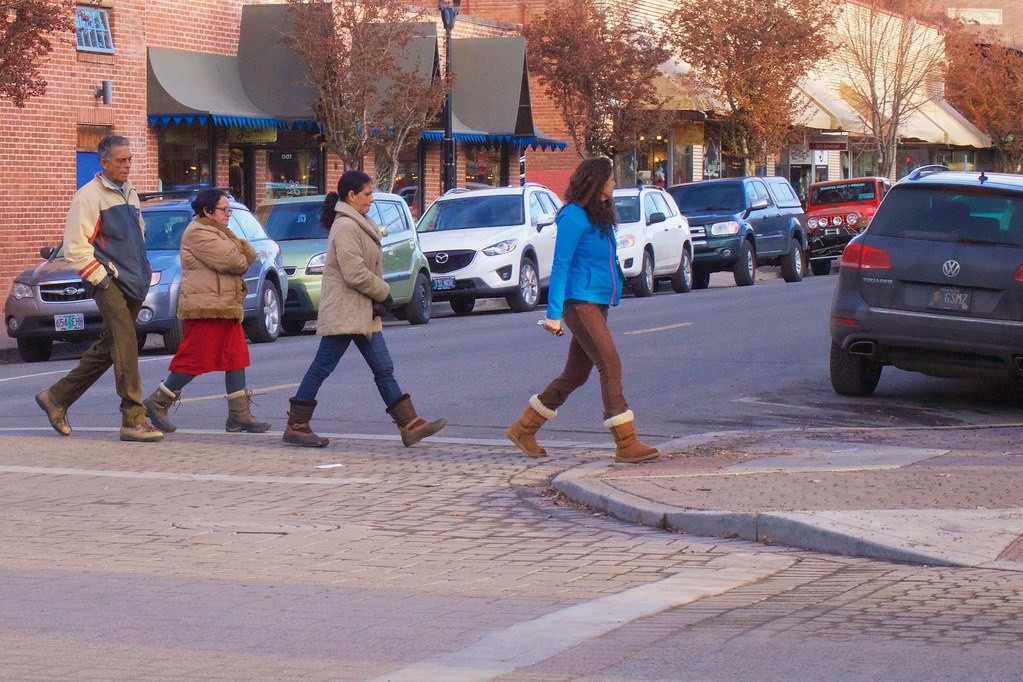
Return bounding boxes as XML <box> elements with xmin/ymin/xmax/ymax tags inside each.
<box><xmin>119</xmin><ymin>422</ymin><xmax>164</xmax><ymax>442</ymax></box>
<box><xmin>34</xmin><ymin>387</ymin><xmax>72</xmax><ymax>436</ymax></box>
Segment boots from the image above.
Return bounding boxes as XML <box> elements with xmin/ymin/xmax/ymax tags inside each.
<box><xmin>224</xmin><ymin>387</ymin><xmax>272</xmax><ymax>433</ymax></box>
<box><xmin>141</xmin><ymin>379</ymin><xmax>182</xmax><ymax>433</ymax></box>
<box><xmin>386</xmin><ymin>393</ymin><xmax>447</xmax><ymax>448</ymax></box>
<box><xmin>604</xmin><ymin>409</ymin><xmax>660</xmax><ymax>463</ymax></box>
<box><xmin>504</xmin><ymin>394</ymin><xmax>558</xmax><ymax>457</ymax></box>
<box><xmin>283</xmin><ymin>397</ymin><xmax>329</xmax><ymax>447</ymax></box>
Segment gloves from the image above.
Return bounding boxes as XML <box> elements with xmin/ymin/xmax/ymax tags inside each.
<box><xmin>373</xmin><ymin>292</ymin><xmax>394</xmax><ymax>316</ymax></box>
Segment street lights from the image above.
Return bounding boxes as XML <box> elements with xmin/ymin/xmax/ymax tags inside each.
<box><xmin>437</xmin><ymin>0</ymin><xmax>458</xmax><ymax>192</ymax></box>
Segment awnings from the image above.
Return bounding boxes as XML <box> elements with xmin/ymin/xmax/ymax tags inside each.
<box><xmin>147</xmin><ymin>3</ymin><xmax>567</xmax><ymax>153</ymax></box>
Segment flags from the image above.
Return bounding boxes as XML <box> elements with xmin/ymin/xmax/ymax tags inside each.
<box><xmin>604</xmin><ymin>30</ymin><xmax>994</xmax><ymax>148</ymax></box>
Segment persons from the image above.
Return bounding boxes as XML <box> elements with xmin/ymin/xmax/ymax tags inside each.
<box><xmin>282</xmin><ymin>171</ymin><xmax>446</xmax><ymax>447</ymax></box>
<box><xmin>142</xmin><ymin>188</ymin><xmax>271</xmax><ymax>433</ymax></box>
<box><xmin>35</xmin><ymin>135</ymin><xmax>164</xmax><ymax>443</ymax></box>
<box><xmin>230</xmin><ymin>149</ymin><xmax>245</xmax><ymax>204</ymax></box>
<box><xmin>506</xmin><ymin>157</ymin><xmax>660</xmax><ymax>466</ymax></box>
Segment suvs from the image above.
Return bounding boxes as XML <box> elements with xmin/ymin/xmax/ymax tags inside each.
<box><xmin>829</xmin><ymin>164</ymin><xmax>1022</xmax><ymax>409</ymax></box>
<box><xmin>416</xmin><ymin>181</ymin><xmax>567</xmax><ymax>315</ymax></box>
<box><xmin>664</xmin><ymin>175</ymin><xmax>813</xmax><ymax>288</ymax></box>
<box><xmin>4</xmin><ymin>187</ymin><xmax>288</xmax><ymax>362</ymax></box>
<box><xmin>804</xmin><ymin>176</ymin><xmax>894</xmax><ymax>276</ymax></box>
<box><xmin>610</xmin><ymin>185</ymin><xmax>694</xmax><ymax>299</ymax></box>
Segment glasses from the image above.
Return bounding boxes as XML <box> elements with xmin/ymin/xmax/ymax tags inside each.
<box><xmin>215</xmin><ymin>206</ymin><xmax>233</xmax><ymax>214</ymax></box>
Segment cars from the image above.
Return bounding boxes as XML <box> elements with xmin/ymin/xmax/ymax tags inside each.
<box><xmin>251</xmin><ymin>192</ymin><xmax>433</xmax><ymax>333</ymax></box>
